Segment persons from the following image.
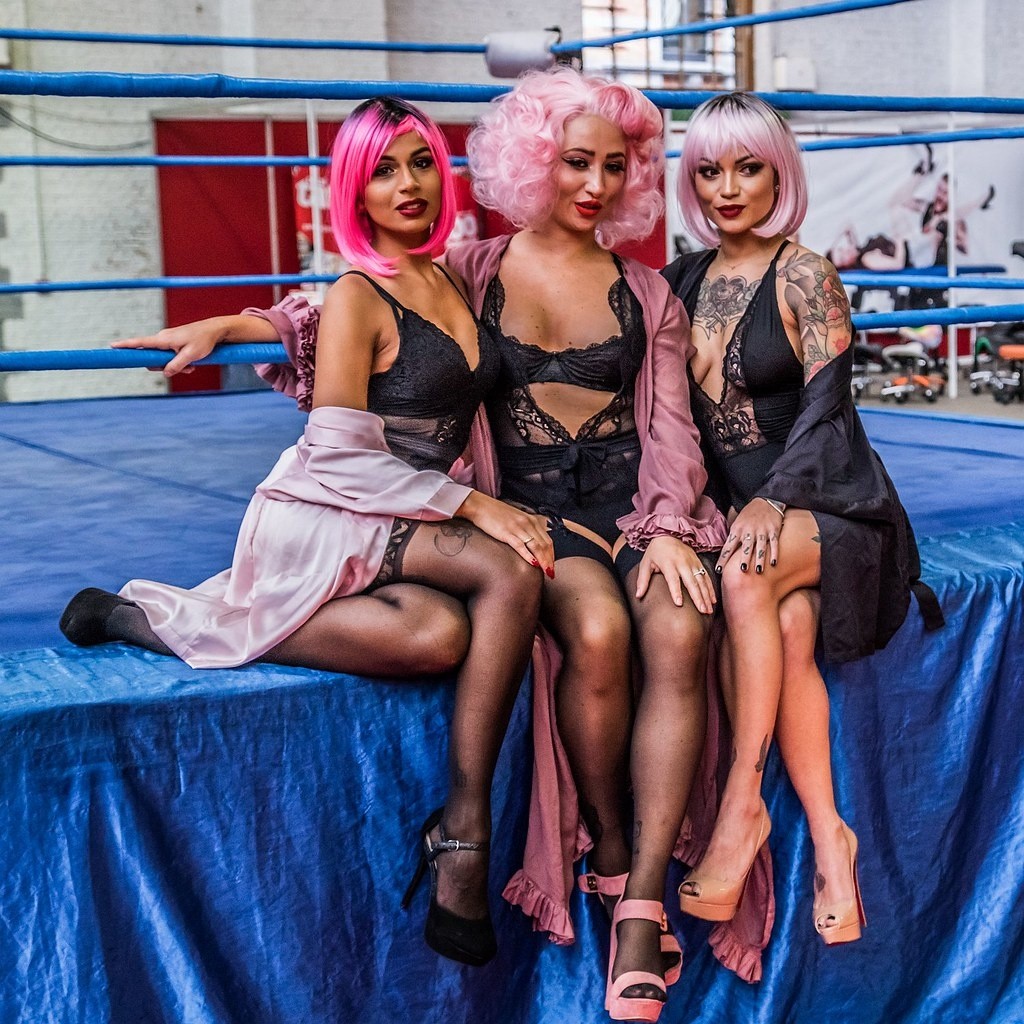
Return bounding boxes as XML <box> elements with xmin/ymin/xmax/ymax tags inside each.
<box><xmin>827</xmin><ymin>140</ymin><xmax>996</xmax><ymax>273</ymax></box>
<box><xmin>653</xmin><ymin>87</ymin><xmax>952</xmax><ymax>950</ymax></box>
<box><xmin>61</xmin><ymin>94</ymin><xmax>557</xmax><ymax>967</ymax></box>
<box><xmin>896</xmin><ymin>159</ymin><xmax>969</xmax><ymax>310</ymax></box>
<box><xmin>113</xmin><ymin>59</ymin><xmax>734</xmax><ymax>1024</ymax></box>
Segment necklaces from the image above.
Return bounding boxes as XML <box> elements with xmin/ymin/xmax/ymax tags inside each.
<box><xmin>719</xmin><ymin>238</ymin><xmax>780</xmax><ymax>270</ymax></box>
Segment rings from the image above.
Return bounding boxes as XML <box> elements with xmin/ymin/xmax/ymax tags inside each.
<box><xmin>524</xmin><ymin>536</ymin><xmax>534</xmax><ymax>543</ymax></box>
<box><xmin>693</xmin><ymin>568</ymin><xmax>706</xmax><ymax>577</ymax></box>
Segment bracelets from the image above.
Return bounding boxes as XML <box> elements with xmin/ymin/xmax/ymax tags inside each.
<box><xmin>760</xmin><ymin>496</ymin><xmax>786</xmax><ymax>519</ymax></box>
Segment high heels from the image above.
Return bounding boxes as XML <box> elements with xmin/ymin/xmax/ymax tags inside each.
<box><xmin>59</xmin><ymin>586</ymin><xmax>141</xmax><ymax>647</ymax></box>
<box><xmin>579</xmin><ymin>869</ymin><xmax>684</xmax><ymax>987</ymax></box>
<box><xmin>812</xmin><ymin>816</ymin><xmax>867</xmax><ymax>947</ymax></box>
<box><xmin>401</xmin><ymin>805</ymin><xmax>498</xmax><ymax>968</ymax></box>
<box><xmin>602</xmin><ymin>884</ymin><xmax>668</xmax><ymax>1024</ymax></box>
<box><xmin>676</xmin><ymin>796</ymin><xmax>772</xmax><ymax>923</ymax></box>
